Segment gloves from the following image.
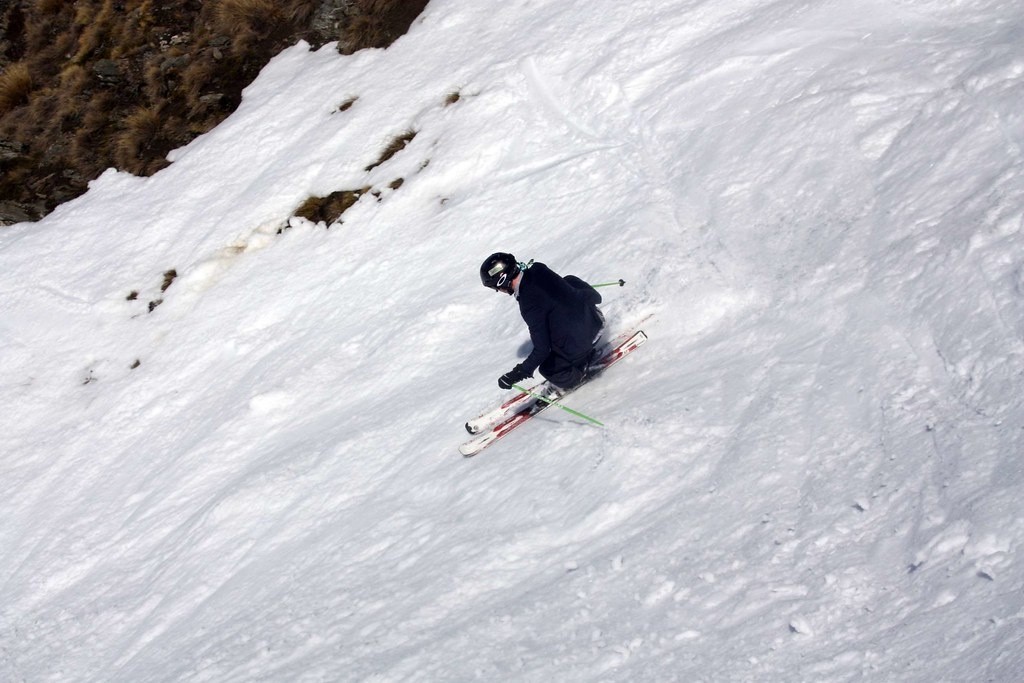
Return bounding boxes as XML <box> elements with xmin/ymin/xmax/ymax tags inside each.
<box><xmin>498</xmin><ymin>371</ymin><xmax>520</xmax><ymax>389</ymax></box>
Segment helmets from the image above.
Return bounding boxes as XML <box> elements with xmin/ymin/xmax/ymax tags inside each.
<box><xmin>480</xmin><ymin>253</ymin><xmax>516</xmax><ymax>290</ymax></box>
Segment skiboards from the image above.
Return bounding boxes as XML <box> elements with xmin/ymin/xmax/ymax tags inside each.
<box><xmin>457</xmin><ymin>313</ymin><xmax>660</xmax><ymax>458</ymax></box>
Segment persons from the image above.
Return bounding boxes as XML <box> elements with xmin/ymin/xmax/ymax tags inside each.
<box><xmin>480</xmin><ymin>252</ymin><xmax>606</xmax><ymax>390</ymax></box>
